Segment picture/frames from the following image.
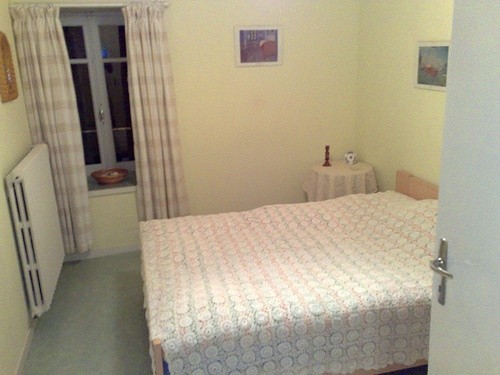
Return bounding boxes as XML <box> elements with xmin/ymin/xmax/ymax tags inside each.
<box><xmin>235</xmin><ymin>25</ymin><xmax>283</xmax><ymax>66</ymax></box>
<box><xmin>413</xmin><ymin>41</ymin><xmax>450</xmax><ymax>91</ymax></box>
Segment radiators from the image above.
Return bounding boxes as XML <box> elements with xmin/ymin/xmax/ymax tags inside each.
<box><xmin>4</xmin><ymin>142</ymin><xmax>66</xmax><ymax>319</ymax></box>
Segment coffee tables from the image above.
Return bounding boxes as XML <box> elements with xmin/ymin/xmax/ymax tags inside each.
<box><xmin>302</xmin><ymin>160</ymin><xmax>378</xmax><ymax>202</ymax></box>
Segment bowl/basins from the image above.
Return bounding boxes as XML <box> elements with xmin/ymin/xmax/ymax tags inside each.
<box><xmin>92</xmin><ymin>168</ymin><xmax>128</xmax><ymax>184</ymax></box>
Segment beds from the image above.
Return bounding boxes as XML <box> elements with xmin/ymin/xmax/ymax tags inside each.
<box><xmin>138</xmin><ymin>190</ymin><xmax>438</xmax><ymax>375</ymax></box>
